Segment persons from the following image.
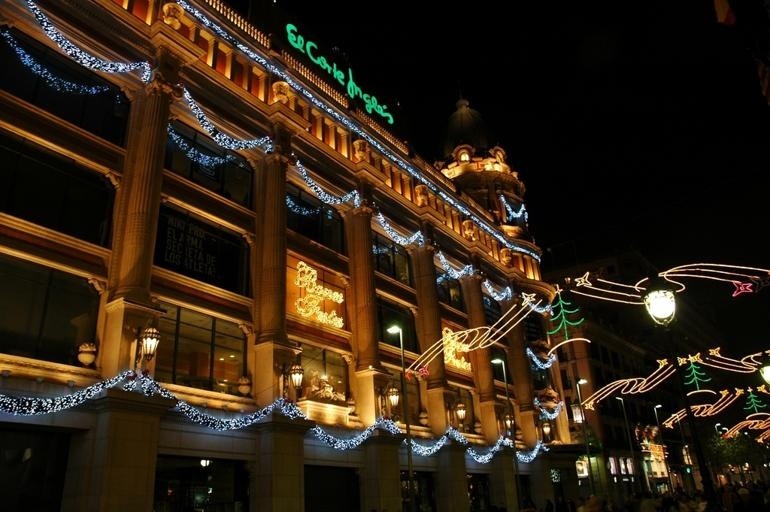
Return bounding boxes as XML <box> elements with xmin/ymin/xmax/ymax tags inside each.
<box><xmin>545</xmin><ymin>479</ymin><xmax>768</xmax><ymax>512</ymax></box>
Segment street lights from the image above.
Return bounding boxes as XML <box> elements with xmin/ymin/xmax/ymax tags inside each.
<box><xmin>653</xmin><ymin>404</ymin><xmax>676</xmax><ymax>496</ymax></box>
<box><xmin>714</xmin><ymin>422</ymin><xmax>729</xmax><ymax>436</ymax></box>
<box><xmin>757</xmin><ymin>351</ymin><xmax>770</xmax><ymax>391</ymax></box>
<box><xmin>614</xmin><ymin>395</ymin><xmax>642</xmax><ymax>494</ymax></box>
<box><xmin>639</xmin><ymin>266</ymin><xmax>722</xmax><ymax>512</ymax></box>
<box><xmin>577</xmin><ymin>377</ymin><xmax>598</xmax><ymax>498</ymax></box>
<box><xmin>385</xmin><ymin>319</ymin><xmax>419</xmax><ymax>512</ymax></box>
<box><xmin>489</xmin><ymin>357</ymin><xmax>524</xmax><ymax>512</ymax></box>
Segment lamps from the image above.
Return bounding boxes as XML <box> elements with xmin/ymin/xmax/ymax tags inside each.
<box><xmin>134</xmin><ymin>317</ymin><xmax>161</xmax><ymax>371</ymax></box>
<box><xmin>542</xmin><ymin>422</ymin><xmax>551</xmax><ymax>435</ymax></box>
<box><xmin>282</xmin><ymin>354</ymin><xmax>303</xmax><ymax>390</ymax></box>
<box><xmin>505</xmin><ymin>414</ymin><xmax>515</xmax><ymax>431</ymax></box>
<box><xmin>378</xmin><ymin>381</ymin><xmax>399</xmax><ymax>407</ymax></box>
<box><xmin>448</xmin><ymin>398</ymin><xmax>466</xmax><ymax>424</ymax></box>
